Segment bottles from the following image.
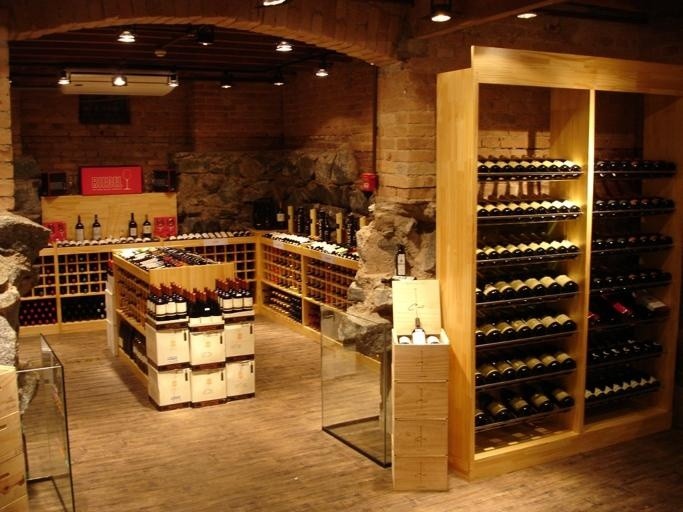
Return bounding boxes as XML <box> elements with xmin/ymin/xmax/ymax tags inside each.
<box><xmin>18</xmin><ymin>215</ymin><xmax>254</xmax><ymax>372</ymax></box>
<box><xmin>144</xmin><ymin>170</ymin><xmax>158</xmax><ymax>192</ymax></box>
<box><xmin>476</xmin><ymin>156</ymin><xmax>678</xmax><ymax>430</ymax></box>
<box><xmin>396</xmin><ymin>243</ymin><xmax>408</xmax><ymax>276</ymax></box>
<box><xmin>65</xmin><ymin>175</ymin><xmax>79</xmax><ymax>196</ymax></box>
<box><xmin>262</xmin><ymin>202</ymin><xmax>359</xmax><ymax>322</ymax></box>
<box><xmin>399</xmin><ymin>317</ymin><xmax>441</xmax><ymax>347</ymax></box>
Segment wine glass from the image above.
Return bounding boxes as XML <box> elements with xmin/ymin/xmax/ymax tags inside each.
<box><xmin>121</xmin><ymin>169</ymin><xmax>132</xmax><ymax>191</ymax></box>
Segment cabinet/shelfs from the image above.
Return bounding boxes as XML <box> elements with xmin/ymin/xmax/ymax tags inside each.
<box><xmin>14</xmin><ymin>244</ymin><xmax>60</xmax><ymax>338</ymax></box>
<box><xmin>433</xmin><ymin>39</ymin><xmax>683</xmax><ymax>484</ymax></box>
<box><xmin>256</xmin><ymin>232</ymin><xmax>303</xmax><ymax>333</ymax></box>
<box><xmin>164</xmin><ymin>230</ymin><xmax>261</xmax><ymax>317</ymax></box>
<box><xmin>53</xmin><ymin>243</ymin><xmax>108</xmax><ymax>328</ymax></box>
<box><xmin>110</xmin><ymin>244</ymin><xmax>240</xmax><ymax>399</ymax></box>
<box><xmin>301</xmin><ymin>246</ymin><xmax>381</xmax><ymax>365</ymax></box>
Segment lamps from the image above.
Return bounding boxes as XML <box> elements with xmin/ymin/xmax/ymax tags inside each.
<box><xmin>47</xmin><ymin>22</ymin><xmax>331</xmax><ymax>92</ymax></box>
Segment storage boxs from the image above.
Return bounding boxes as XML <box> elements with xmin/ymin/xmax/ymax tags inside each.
<box><xmin>390</xmin><ymin>328</ymin><xmax>450</xmax><ymax>494</ymax></box>
<box><xmin>0</xmin><ymin>363</ymin><xmax>34</xmax><ymax>511</ymax></box>
<box><xmin>141</xmin><ymin>305</ymin><xmax>257</xmax><ymax>412</ymax></box>
